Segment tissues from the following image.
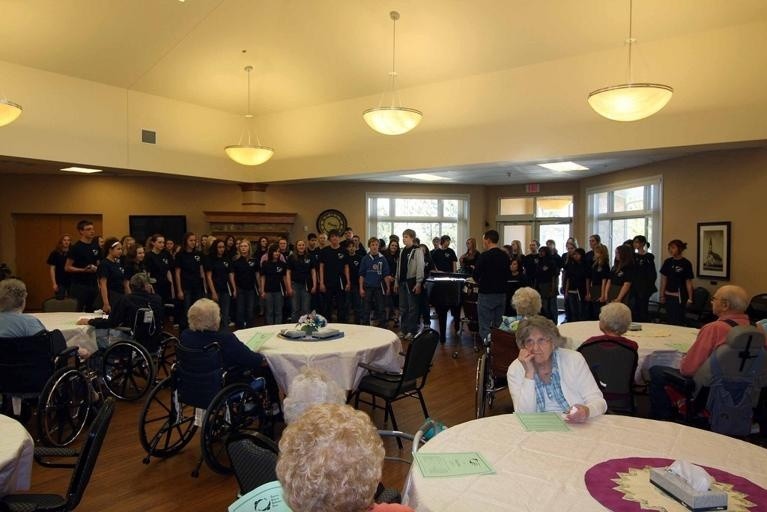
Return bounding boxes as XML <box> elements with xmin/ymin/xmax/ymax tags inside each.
<box><xmin>649</xmin><ymin>458</ymin><xmax>728</xmax><ymax>511</ymax></box>
<box><xmin>311</xmin><ymin>327</ymin><xmax>339</xmax><ymax>340</ymax></box>
<box><xmin>628</xmin><ymin>323</ymin><xmax>642</xmax><ymax>331</ymax></box>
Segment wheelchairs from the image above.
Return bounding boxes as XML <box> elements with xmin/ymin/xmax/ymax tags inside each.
<box><xmin>0</xmin><ymin>329</ymin><xmax>111</xmax><ymax>453</ymax></box>
<box><xmin>474</xmin><ymin>321</ymin><xmax>528</xmax><ymax>423</ymax></box>
<box><xmin>138</xmin><ymin>334</ymin><xmax>274</xmax><ymax>477</ymax></box>
<box><xmin>95</xmin><ymin>303</ymin><xmax>181</xmax><ymax>403</ymax></box>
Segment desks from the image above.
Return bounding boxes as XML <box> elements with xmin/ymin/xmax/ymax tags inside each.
<box><xmin>228</xmin><ymin>321</ymin><xmax>399</xmax><ymax>430</ymax></box>
<box><xmin>1</xmin><ymin>411</ymin><xmax>33</xmax><ymax>498</ymax></box>
<box><xmin>7</xmin><ymin>312</ymin><xmax>112</xmax><ymax>360</ymax></box>
<box><xmin>404</xmin><ymin>409</ymin><xmax>766</xmax><ymax>511</ymax></box>
<box><xmin>557</xmin><ymin>321</ymin><xmax>701</xmax><ymax>405</ymax></box>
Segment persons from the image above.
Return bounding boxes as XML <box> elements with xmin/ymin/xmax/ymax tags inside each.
<box><xmin>647</xmin><ymin>284</ymin><xmax>766</xmax><ymax>421</ymax></box>
<box><xmin>279</xmin><ymin>363</ymin><xmax>350</xmax><ymax>425</ymax></box>
<box><xmin>1</xmin><ymin>277</ymin><xmax>107</xmax><ymax>412</ymax></box>
<box><xmin>45</xmin><ymin>221</ymin><xmax>695</xmax><ymax>341</ymax></box>
<box><xmin>275</xmin><ymin>401</ymin><xmax>417</xmax><ymax>512</ymax></box>
<box><xmin>502</xmin><ymin>316</ymin><xmax>608</xmax><ymax>424</ymax></box>
<box><xmin>176</xmin><ymin>296</ymin><xmax>280</xmax><ymax>419</ymax></box>
<box><xmin>575</xmin><ymin>301</ymin><xmax>639</xmax><ymax>411</ymax></box>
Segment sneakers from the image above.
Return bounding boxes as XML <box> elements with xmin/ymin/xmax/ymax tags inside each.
<box><xmin>399</xmin><ymin>324</ymin><xmax>430</xmax><ymax>341</ymax></box>
<box><xmin>430</xmin><ymin>312</ymin><xmax>438</xmax><ymax>319</ymax></box>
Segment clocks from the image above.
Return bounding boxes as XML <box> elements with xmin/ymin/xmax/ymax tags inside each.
<box><xmin>316</xmin><ymin>208</ymin><xmax>348</xmax><ymax>237</ymax></box>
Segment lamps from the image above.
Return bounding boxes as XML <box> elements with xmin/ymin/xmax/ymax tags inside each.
<box><xmin>0</xmin><ymin>99</ymin><xmax>23</xmax><ymax>127</ymax></box>
<box><xmin>223</xmin><ymin>66</ymin><xmax>275</xmax><ymax>167</ymax></box>
<box><xmin>587</xmin><ymin>1</ymin><xmax>673</xmax><ymax>123</ymax></box>
<box><xmin>361</xmin><ymin>11</ymin><xmax>423</xmax><ymax>137</ymax></box>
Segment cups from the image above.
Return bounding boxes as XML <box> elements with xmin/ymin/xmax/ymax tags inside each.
<box><xmin>94</xmin><ymin>309</ymin><xmax>107</xmax><ymax>320</ymax></box>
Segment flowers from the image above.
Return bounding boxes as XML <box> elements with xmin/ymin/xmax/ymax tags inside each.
<box><xmin>295</xmin><ymin>313</ymin><xmax>328</xmax><ymax>329</ymax></box>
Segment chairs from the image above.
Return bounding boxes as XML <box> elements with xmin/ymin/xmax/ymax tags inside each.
<box><xmin>354</xmin><ymin>327</ymin><xmax>440</xmax><ymax>449</ymax></box>
<box><xmin>576</xmin><ymin>338</ymin><xmax>638</xmax><ymax>416</ymax></box>
<box><xmin>649</xmin><ymin>324</ymin><xmax>765</xmax><ymax>438</ymax></box>
<box><xmin>1</xmin><ymin>394</ymin><xmax>118</xmax><ymax>511</ymax></box>
<box><xmin>223</xmin><ymin>431</ymin><xmax>288</xmax><ymax>508</ymax></box>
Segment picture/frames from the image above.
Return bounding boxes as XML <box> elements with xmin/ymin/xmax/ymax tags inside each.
<box><xmin>696</xmin><ymin>220</ymin><xmax>732</xmax><ymax>281</ymax></box>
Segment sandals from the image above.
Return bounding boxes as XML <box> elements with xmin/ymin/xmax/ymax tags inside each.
<box><xmin>391</xmin><ymin>317</ymin><xmax>399</xmax><ymax>324</ymax></box>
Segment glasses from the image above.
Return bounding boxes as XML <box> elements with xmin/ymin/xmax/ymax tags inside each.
<box><xmin>526</xmin><ymin>337</ymin><xmax>548</xmax><ymax>346</ymax></box>
<box><xmin>710</xmin><ymin>297</ymin><xmax>717</xmax><ymax>303</ymax></box>
